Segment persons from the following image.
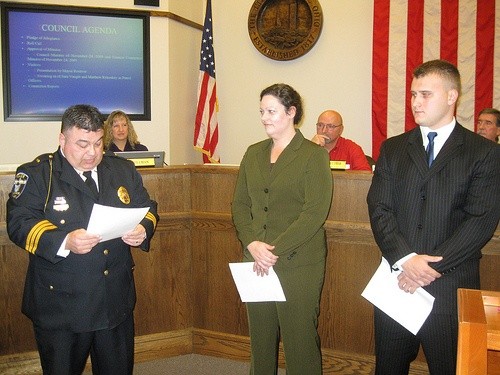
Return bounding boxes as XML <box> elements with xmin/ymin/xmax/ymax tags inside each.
<box><xmin>230</xmin><ymin>83</ymin><xmax>336</xmax><ymax>375</ymax></box>
<box><xmin>364</xmin><ymin>60</ymin><xmax>500</xmax><ymax>375</ymax></box>
<box><xmin>4</xmin><ymin>104</ymin><xmax>160</xmax><ymax>374</ymax></box>
<box><xmin>308</xmin><ymin>110</ymin><xmax>375</xmax><ymax>174</ymax></box>
<box><xmin>476</xmin><ymin>108</ymin><xmax>500</xmax><ymax>142</ymax></box>
<box><xmin>100</xmin><ymin>110</ymin><xmax>151</xmax><ymax>158</ymax></box>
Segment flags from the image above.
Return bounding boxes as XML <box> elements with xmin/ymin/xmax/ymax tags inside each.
<box><xmin>191</xmin><ymin>1</ymin><xmax>221</xmax><ymax>165</ymax></box>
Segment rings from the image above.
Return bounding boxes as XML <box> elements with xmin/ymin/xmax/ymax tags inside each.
<box><xmin>136</xmin><ymin>241</ymin><xmax>138</xmax><ymax>243</ymax></box>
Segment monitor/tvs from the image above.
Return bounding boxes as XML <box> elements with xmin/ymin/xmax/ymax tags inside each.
<box><xmin>0</xmin><ymin>0</ymin><xmax>151</xmax><ymax>121</ymax></box>
<box><xmin>113</xmin><ymin>151</ymin><xmax>165</xmax><ymax>167</ymax></box>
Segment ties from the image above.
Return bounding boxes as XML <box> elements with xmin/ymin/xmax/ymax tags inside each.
<box><xmin>83</xmin><ymin>170</ymin><xmax>99</xmax><ymax>202</ymax></box>
<box><xmin>426</xmin><ymin>132</ymin><xmax>438</xmax><ymax>168</ymax></box>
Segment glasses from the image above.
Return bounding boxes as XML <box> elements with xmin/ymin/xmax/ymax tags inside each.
<box><xmin>316</xmin><ymin>122</ymin><xmax>342</xmax><ymax>130</ymax></box>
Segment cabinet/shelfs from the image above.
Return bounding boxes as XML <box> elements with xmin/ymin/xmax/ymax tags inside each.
<box><xmin>454</xmin><ymin>287</ymin><xmax>500</xmax><ymax>375</ymax></box>
